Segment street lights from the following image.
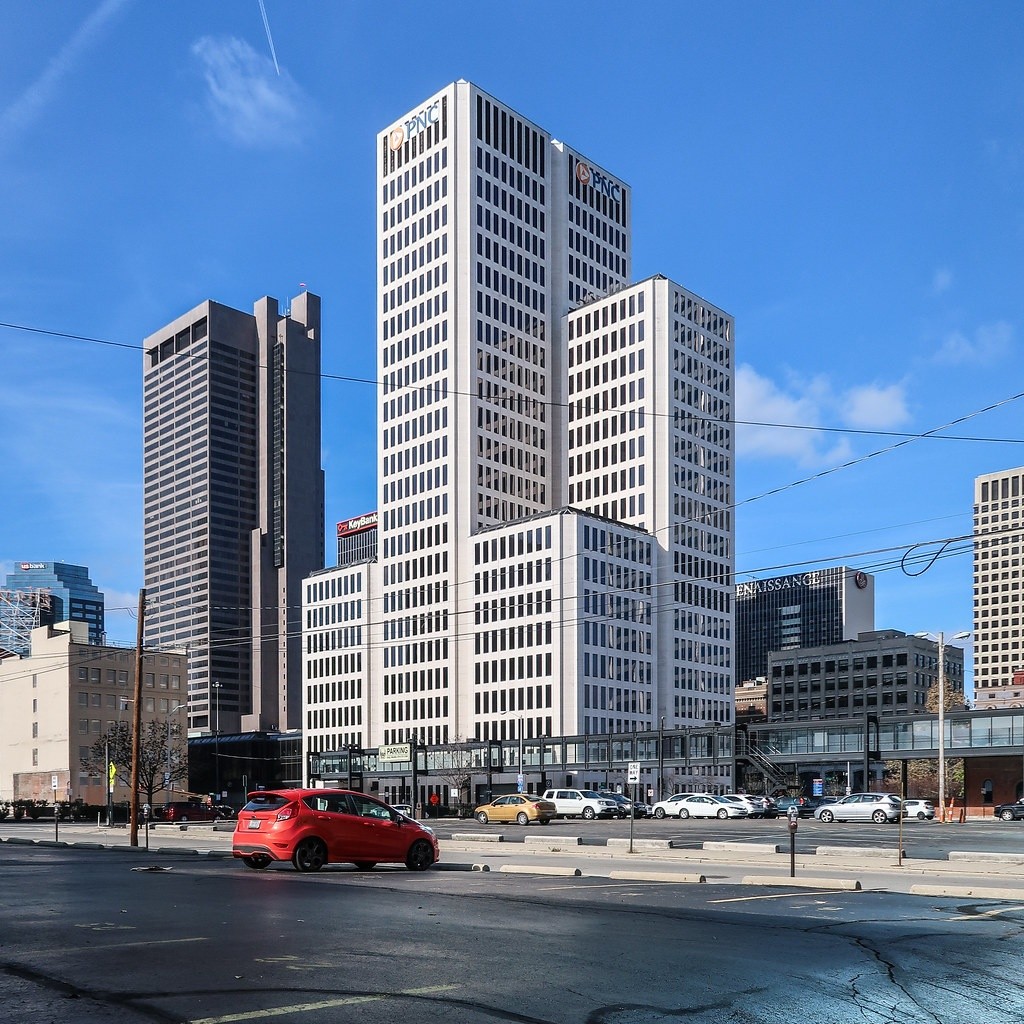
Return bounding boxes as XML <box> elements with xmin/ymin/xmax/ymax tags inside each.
<box><xmin>914</xmin><ymin>629</ymin><xmax>971</xmax><ymax>821</ymax></box>
<box><xmin>660</xmin><ymin>715</ymin><xmax>666</xmax><ymax>802</ymax></box>
<box><xmin>499</xmin><ymin>711</ymin><xmax>522</xmax><ymax>795</ymax></box>
<box><xmin>165</xmin><ymin>704</ymin><xmax>186</xmax><ymax>823</ymax></box>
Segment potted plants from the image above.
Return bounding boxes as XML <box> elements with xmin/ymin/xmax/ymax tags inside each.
<box><xmin>11</xmin><ymin>799</ymin><xmax>29</xmax><ymax>820</ymax></box>
<box><xmin>0</xmin><ymin>797</ymin><xmax>10</xmax><ymax>818</ymax></box>
<box><xmin>28</xmin><ymin>798</ymin><xmax>50</xmax><ymax>820</ymax></box>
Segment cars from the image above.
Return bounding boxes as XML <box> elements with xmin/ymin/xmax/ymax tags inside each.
<box><xmin>994</xmin><ymin>796</ymin><xmax>1024</xmax><ymax>822</ymax></box>
<box><xmin>813</xmin><ymin>793</ymin><xmax>908</xmax><ymax>824</ymax></box>
<box><xmin>232</xmin><ymin>786</ymin><xmax>439</xmax><ymax>873</ymax></box>
<box><xmin>474</xmin><ymin>793</ymin><xmax>556</xmax><ymax>825</ymax></box>
<box><xmin>901</xmin><ymin>799</ymin><xmax>935</xmax><ymax>820</ymax></box>
<box><xmin>648</xmin><ymin>791</ymin><xmax>846</xmax><ymax>820</ymax></box>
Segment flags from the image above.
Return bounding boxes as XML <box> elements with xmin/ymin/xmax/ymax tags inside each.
<box><xmin>517</xmin><ymin>774</ymin><xmax>528</xmax><ymax>793</ymax></box>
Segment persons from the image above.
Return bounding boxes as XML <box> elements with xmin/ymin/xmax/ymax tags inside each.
<box><xmin>761</xmin><ymin>797</ymin><xmax>769</xmax><ymax>818</ymax></box>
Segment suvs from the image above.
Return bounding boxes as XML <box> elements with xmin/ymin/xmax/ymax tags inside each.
<box><xmin>542</xmin><ymin>789</ymin><xmax>619</xmax><ymax>820</ymax></box>
<box><xmin>595</xmin><ymin>790</ymin><xmax>646</xmax><ymax>820</ymax></box>
